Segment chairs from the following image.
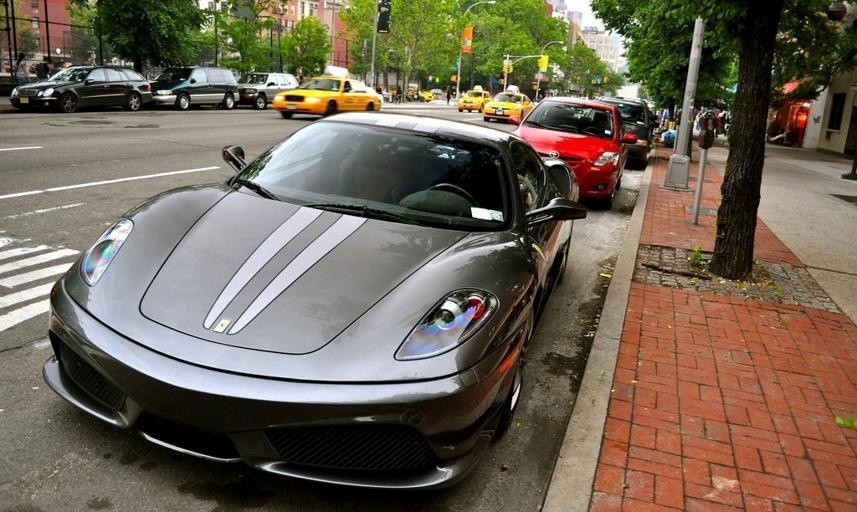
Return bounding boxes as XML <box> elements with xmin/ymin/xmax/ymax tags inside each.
<box><xmin>540</xmin><ymin>109</ymin><xmax>610</xmax><ymax>138</ymax></box>
<box><xmin>318</xmin><ymin>137</ymin><xmax>504</xmax><ymax>222</ymax></box>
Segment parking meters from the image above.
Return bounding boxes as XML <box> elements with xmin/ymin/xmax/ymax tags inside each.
<box><xmin>670</xmin><ymin>108</ymin><xmax>681</xmax><ymax>155</ymax></box>
<box><xmin>689</xmin><ymin>111</ymin><xmax>720</xmax><ymax>227</ymax></box>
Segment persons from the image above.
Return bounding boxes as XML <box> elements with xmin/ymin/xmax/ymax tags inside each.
<box><xmin>655</xmin><ymin>105</ymin><xmax>731</xmax><ymax>140</ymax></box>
<box><xmin>14</xmin><ymin>53</ymin><xmax>97</xmax><ymax>85</ymax></box>
<box><xmin>374</xmin><ymin>83</ymin><xmax>451</xmax><ymax>106</ymax></box>
<box><xmin>767</xmin><ymin>114</ymin><xmax>799</xmax><ymax>144</ymax></box>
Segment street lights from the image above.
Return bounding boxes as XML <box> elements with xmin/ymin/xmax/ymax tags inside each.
<box><xmin>454</xmin><ymin>1</ymin><xmax>498</xmax><ymax>102</ymax></box>
<box><xmin>535</xmin><ymin>39</ymin><xmax>564</xmax><ymax>101</ymax></box>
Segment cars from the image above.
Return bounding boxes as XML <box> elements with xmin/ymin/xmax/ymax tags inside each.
<box><xmin>429</xmin><ymin>88</ymin><xmax>442</xmax><ymax>95</ymax></box>
<box><xmin>455</xmin><ymin>80</ymin><xmax>656</xmax><ymax>210</ymax></box>
<box><xmin>388</xmin><ymin>86</ymin><xmax>432</xmax><ymax>105</ymax></box>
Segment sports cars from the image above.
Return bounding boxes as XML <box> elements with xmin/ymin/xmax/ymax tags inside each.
<box><xmin>42</xmin><ymin>110</ymin><xmax>580</xmax><ymax>492</ymax></box>
